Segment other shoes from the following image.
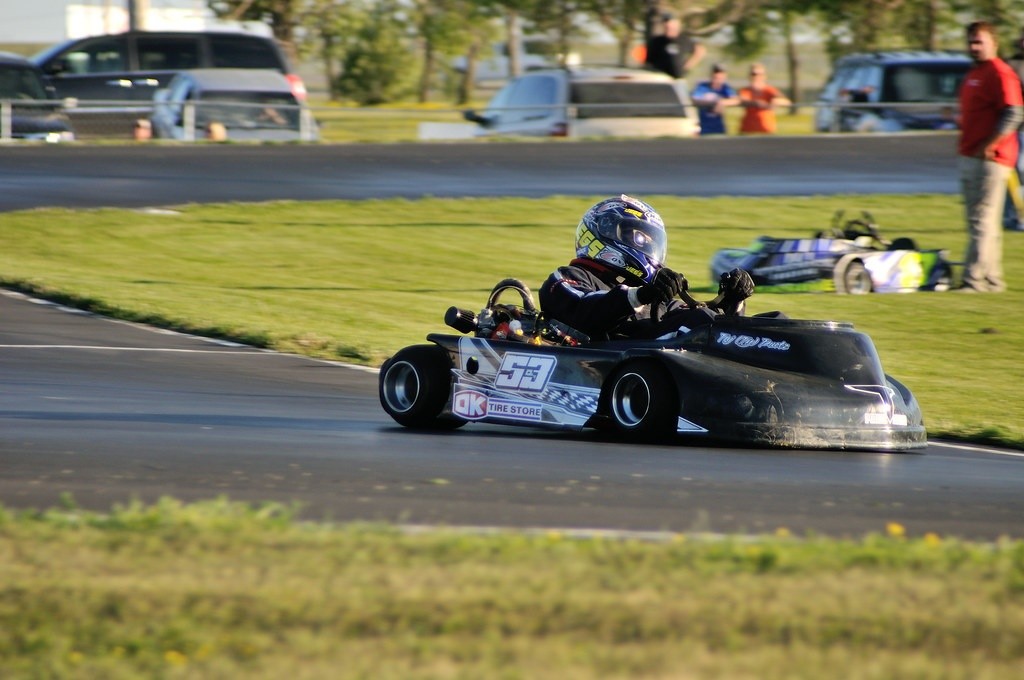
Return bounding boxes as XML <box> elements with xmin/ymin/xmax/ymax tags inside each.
<box><xmin>948</xmin><ymin>279</ymin><xmax>1005</xmax><ymax>295</ymax></box>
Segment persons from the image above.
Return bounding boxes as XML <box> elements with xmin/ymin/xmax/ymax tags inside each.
<box><xmin>739</xmin><ymin>63</ymin><xmax>793</xmax><ymax>132</ymax></box>
<box><xmin>691</xmin><ymin>64</ymin><xmax>741</xmax><ymax>135</ymax></box>
<box><xmin>646</xmin><ymin>15</ymin><xmax>707</xmax><ymax>78</ymax></box>
<box><xmin>539</xmin><ymin>197</ymin><xmax>754</xmax><ymax>346</ymax></box>
<box><xmin>958</xmin><ymin>21</ymin><xmax>1024</xmax><ymax>294</ymax></box>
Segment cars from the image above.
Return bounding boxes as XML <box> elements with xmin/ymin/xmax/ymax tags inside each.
<box><xmin>0</xmin><ymin>50</ymin><xmax>78</xmax><ymax>143</ymax></box>
<box><xmin>29</xmin><ymin>32</ymin><xmax>323</xmax><ymax>145</ymax></box>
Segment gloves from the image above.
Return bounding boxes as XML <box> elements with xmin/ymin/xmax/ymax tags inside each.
<box><xmin>718</xmin><ymin>268</ymin><xmax>755</xmax><ymax>301</ymax></box>
<box><xmin>638</xmin><ymin>267</ymin><xmax>683</xmax><ymax>304</ymax></box>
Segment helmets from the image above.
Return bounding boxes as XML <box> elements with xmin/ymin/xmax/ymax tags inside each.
<box><xmin>575</xmin><ymin>194</ymin><xmax>669</xmax><ymax>285</ymax></box>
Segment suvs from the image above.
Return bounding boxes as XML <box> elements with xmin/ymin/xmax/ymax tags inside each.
<box><xmin>462</xmin><ymin>65</ymin><xmax>700</xmax><ymax>140</ymax></box>
<box><xmin>816</xmin><ymin>54</ymin><xmax>978</xmax><ymax>133</ymax></box>
